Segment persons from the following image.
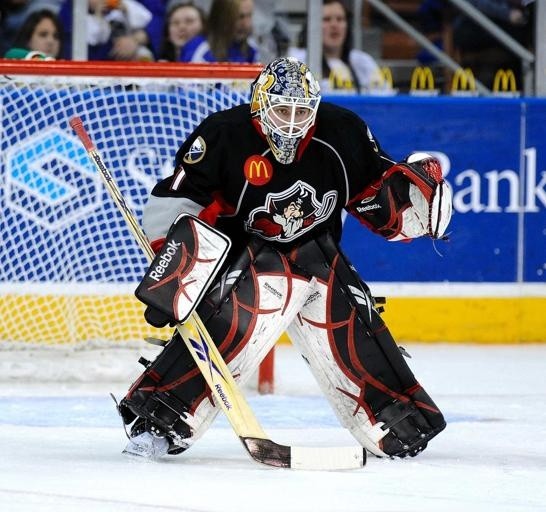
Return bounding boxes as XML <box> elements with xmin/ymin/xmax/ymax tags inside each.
<box><xmin>115</xmin><ymin>58</ymin><xmax>447</xmax><ymax>464</ymax></box>
<box><xmin>1</xmin><ymin>1</ymin><xmax>292</xmax><ymax>66</ymax></box>
<box><xmin>286</xmin><ymin>1</ymin><xmax>393</xmax><ymax>92</ymax></box>
<box><xmin>413</xmin><ymin>1</ymin><xmax>537</xmax><ymax>92</ymax></box>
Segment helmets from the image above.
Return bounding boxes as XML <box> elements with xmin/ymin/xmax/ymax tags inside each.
<box><xmin>251</xmin><ymin>59</ymin><xmax>322</xmax><ymax>164</ymax></box>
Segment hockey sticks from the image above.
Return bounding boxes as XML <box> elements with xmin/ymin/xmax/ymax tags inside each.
<box><xmin>70</xmin><ymin>116</ymin><xmax>366</xmax><ymax>470</ymax></box>
